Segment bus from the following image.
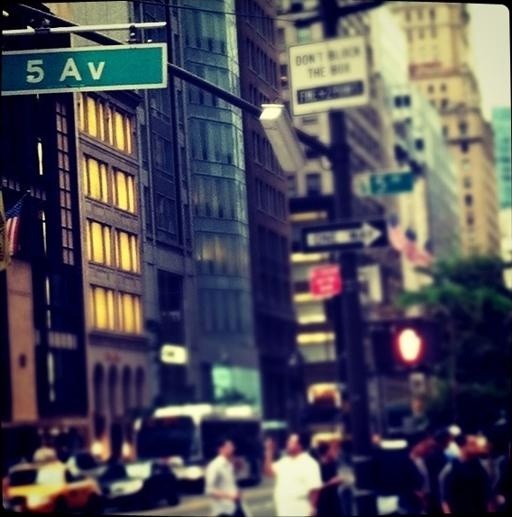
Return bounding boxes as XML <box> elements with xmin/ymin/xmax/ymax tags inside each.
<box><xmin>0</xmin><ymin>416</ymin><xmax>92</xmax><ymax>461</ymax></box>
<box><xmin>132</xmin><ymin>404</ymin><xmax>262</xmax><ymax>486</ymax></box>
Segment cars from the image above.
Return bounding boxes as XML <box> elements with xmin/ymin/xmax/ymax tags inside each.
<box><xmin>2</xmin><ymin>455</ymin><xmax>181</xmax><ymax>515</ymax></box>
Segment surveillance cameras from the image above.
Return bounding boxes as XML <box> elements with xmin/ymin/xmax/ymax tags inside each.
<box><xmin>259</xmin><ymin>102</ymin><xmax>306</xmax><ymax>173</ymax></box>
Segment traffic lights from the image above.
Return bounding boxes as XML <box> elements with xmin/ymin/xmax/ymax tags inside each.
<box><xmin>394</xmin><ymin>318</ymin><xmax>430</xmax><ymax>372</ymax></box>
